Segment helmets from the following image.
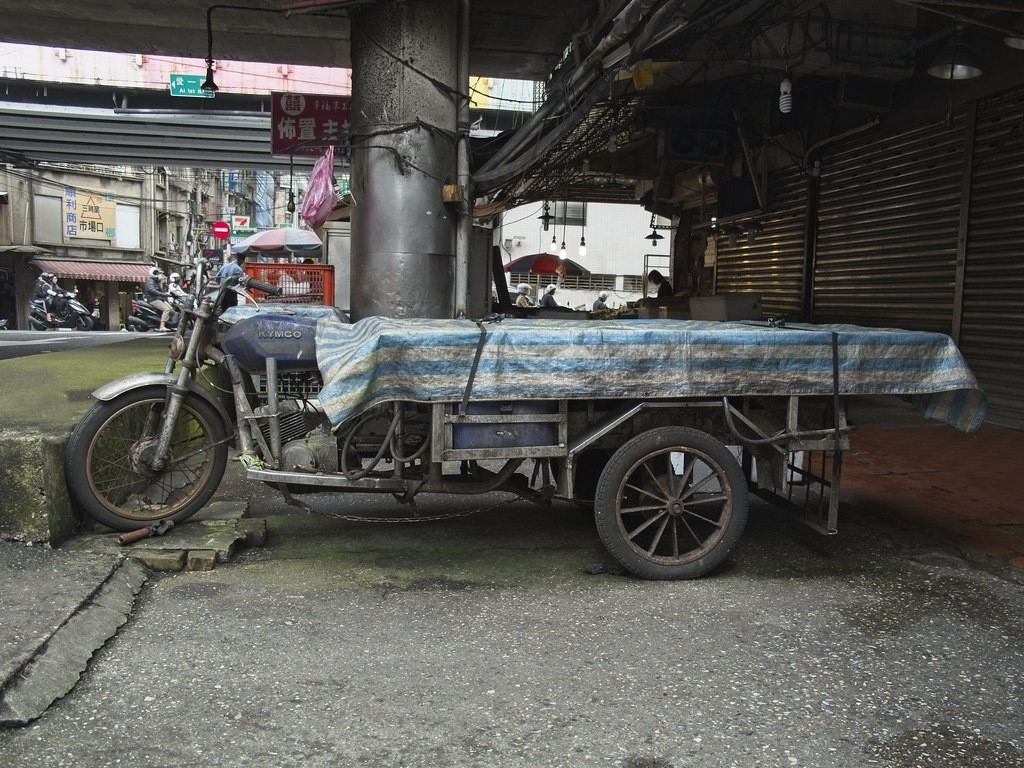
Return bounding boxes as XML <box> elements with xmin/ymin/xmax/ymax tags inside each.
<box><xmin>149</xmin><ymin>267</ymin><xmax>164</xmax><ymax>279</ymax></box>
<box><xmin>170</xmin><ymin>272</ymin><xmax>180</xmax><ymax>282</ymax></box>
<box><xmin>517</xmin><ymin>283</ymin><xmax>532</xmax><ymax>296</ymax></box>
<box><xmin>41</xmin><ymin>272</ymin><xmax>54</xmax><ymax>279</ymax></box>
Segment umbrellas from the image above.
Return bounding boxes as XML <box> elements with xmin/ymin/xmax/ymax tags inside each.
<box><xmin>231</xmin><ymin>227</ymin><xmax>322</xmax><ymax>263</ymax></box>
<box><xmin>504</xmin><ymin>253</ymin><xmax>591</xmax><ymax>293</ymax></box>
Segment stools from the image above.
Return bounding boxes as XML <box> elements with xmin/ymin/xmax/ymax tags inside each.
<box><xmin>119</xmin><ymin>324</ymin><xmax>126</xmax><ymax>330</ymax></box>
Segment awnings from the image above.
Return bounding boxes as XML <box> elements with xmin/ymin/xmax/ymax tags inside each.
<box><xmin>32</xmin><ymin>256</ymin><xmax>164</xmax><ymax>282</ymax></box>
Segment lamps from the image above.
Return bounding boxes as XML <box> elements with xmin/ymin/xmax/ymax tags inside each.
<box><xmin>1002</xmin><ymin>15</ymin><xmax>1024</xmax><ymax>50</ymax></box>
<box><xmin>537</xmin><ymin>211</ymin><xmax>762</xmax><ymax>247</ymax></box>
<box><xmin>513</xmin><ymin>236</ymin><xmax>526</xmax><ymax>247</ymax></box>
<box><xmin>201</xmin><ymin>67</ymin><xmax>218</xmax><ymax>91</ymax></box>
<box><xmin>926</xmin><ymin>21</ymin><xmax>990</xmax><ymax>81</ymax></box>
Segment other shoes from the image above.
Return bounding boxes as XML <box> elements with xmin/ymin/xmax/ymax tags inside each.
<box><xmin>159</xmin><ymin>328</ymin><xmax>171</xmax><ymax>332</ymax></box>
<box><xmin>47</xmin><ymin>316</ymin><xmax>52</xmax><ymax>322</ymax></box>
<box><xmin>55</xmin><ymin>317</ymin><xmax>66</xmax><ymax>323</ymax></box>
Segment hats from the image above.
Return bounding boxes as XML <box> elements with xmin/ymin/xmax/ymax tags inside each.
<box><xmin>235</xmin><ymin>251</ymin><xmax>245</xmax><ymax>259</ymax></box>
<box><xmin>545</xmin><ymin>284</ymin><xmax>556</xmax><ymax>295</ymax></box>
<box><xmin>599</xmin><ymin>290</ymin><xmax>608</xmax><ymax>299</ymax></box>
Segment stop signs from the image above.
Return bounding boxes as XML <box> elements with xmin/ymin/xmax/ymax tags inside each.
<box><xmin>212</xmin><ymin>221</ymin><xmax>230</xmax><ymax>238</ymax></box>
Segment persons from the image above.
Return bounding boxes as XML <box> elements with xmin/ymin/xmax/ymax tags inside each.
<box><xmin>593</xmin><ymin>292</ymin><xmax>608</xmax><ymax>312</ymax></box>
<box><xmin>145</xmin><ymin>266</ymin><xmax>174</xmax><ymax>332</ymax></box>
<box><xmin>215</xmin><ymin>253</ymin><xmax>246</xmax><ymax>312</ymax></box>
<box><xmin>516</xmin><ymin>283</ymin><xmax>540</xmax><ymax>308</ymax></box>
<box><xmin>274</xmin><ymin>258</ymin><xmax>315</xmax><ymax>297</ymax></box>
<box><xmin>168</xmin><ymin>273</ymin><xmax>198</xmax><ymax>331</ymax></box>
<box><xmin>541</xmin><ymin>284</ymin><xmax>559</xmax><ymax>307</ymax></box>
<box><xmin>648</xmin><ymin>270</ymin><xmax>672</xmax><ymax>297</ymax></box>
<box><xmin>31</xmin><ymin>272</ymin><xmax>77</xmax><ymax>322</ymax></box>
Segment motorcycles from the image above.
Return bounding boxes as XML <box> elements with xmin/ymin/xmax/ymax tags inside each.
<box><xmin>63</xmin><ymin>257</ymin><xmax>951</xmax><ymax>579</ymax></box>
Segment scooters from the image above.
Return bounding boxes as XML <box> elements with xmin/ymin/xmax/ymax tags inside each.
<box><xmin>27</xmin><ymin>284</ymin><xmax>93</xmax><ymax>331</ymax></box>
<box><xmin>127</xmin><ymin>289</ymin><xmax>196</xmax><ymax>333</ymax></box>
